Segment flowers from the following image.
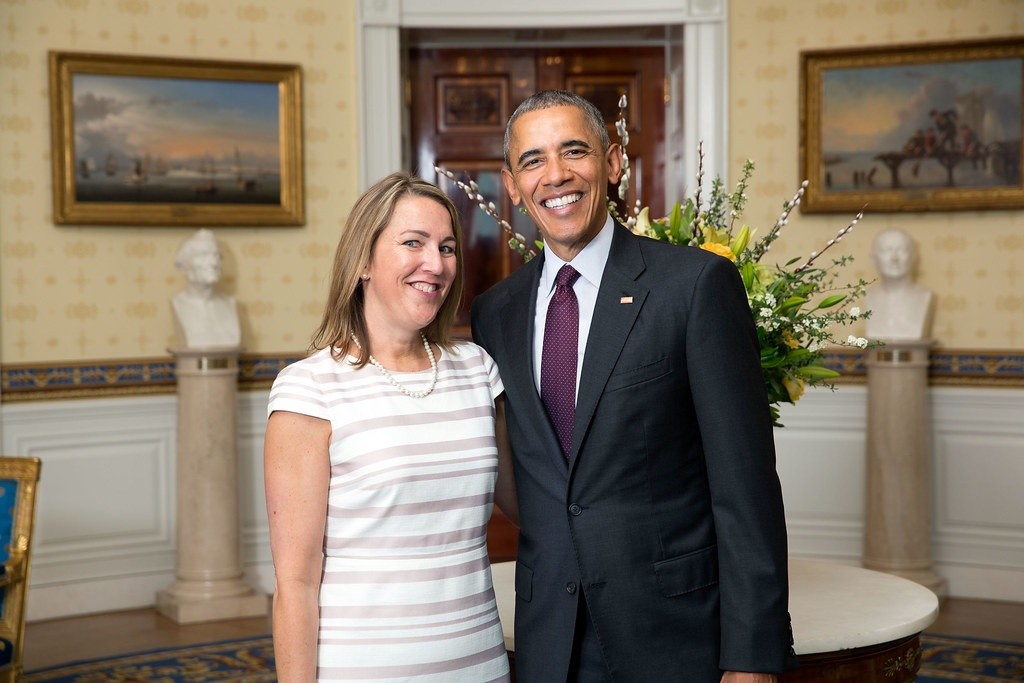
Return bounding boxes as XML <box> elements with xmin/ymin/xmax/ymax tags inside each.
<box><xmin>436</xmin><ymin>94</ymin><xmax>877</xmax><ymax>426</ymax></box>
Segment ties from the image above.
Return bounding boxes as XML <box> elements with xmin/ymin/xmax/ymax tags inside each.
<box><xmin>541</xmin><ymin>265</ymin><xmax>581</xmax><ymax>468</ymax></box>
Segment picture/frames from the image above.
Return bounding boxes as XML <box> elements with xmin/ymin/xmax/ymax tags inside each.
<box><xmin>47</xmin><ymin>47</ymin><xmax>304</xmax><ymax>230</ymax></box>
<box><xmin>797</xmin><ymin>34</ymin><xmax>1024</xmax><ymax>214</ymax></box>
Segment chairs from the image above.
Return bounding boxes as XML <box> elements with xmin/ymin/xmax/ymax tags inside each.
<box><xmin>0</xmin><ymin>455</ymin><xmax>42</xmax><ymax>683</ymax></box>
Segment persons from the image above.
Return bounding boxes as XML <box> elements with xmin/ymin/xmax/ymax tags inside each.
<box><xmin>262</xmin><ymin>173</ymin><xmax>522</xmax><ymax>683</ymax></box>
<box><xmin>469</xmin><ymin>89</ymin><xmax>795</xmax><ymax>683</ymax></box>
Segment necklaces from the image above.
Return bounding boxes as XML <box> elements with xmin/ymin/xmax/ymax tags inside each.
<box><xmin>347</xmin><ymin>323</ymin><xmax>438</xmax><ymax>399</ymax></box>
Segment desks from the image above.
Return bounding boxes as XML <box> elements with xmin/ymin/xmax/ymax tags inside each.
<box><xmin>490</xmin><ymin>560</ymin><xmax>938</xmax><ymax>683</ymax></box>
